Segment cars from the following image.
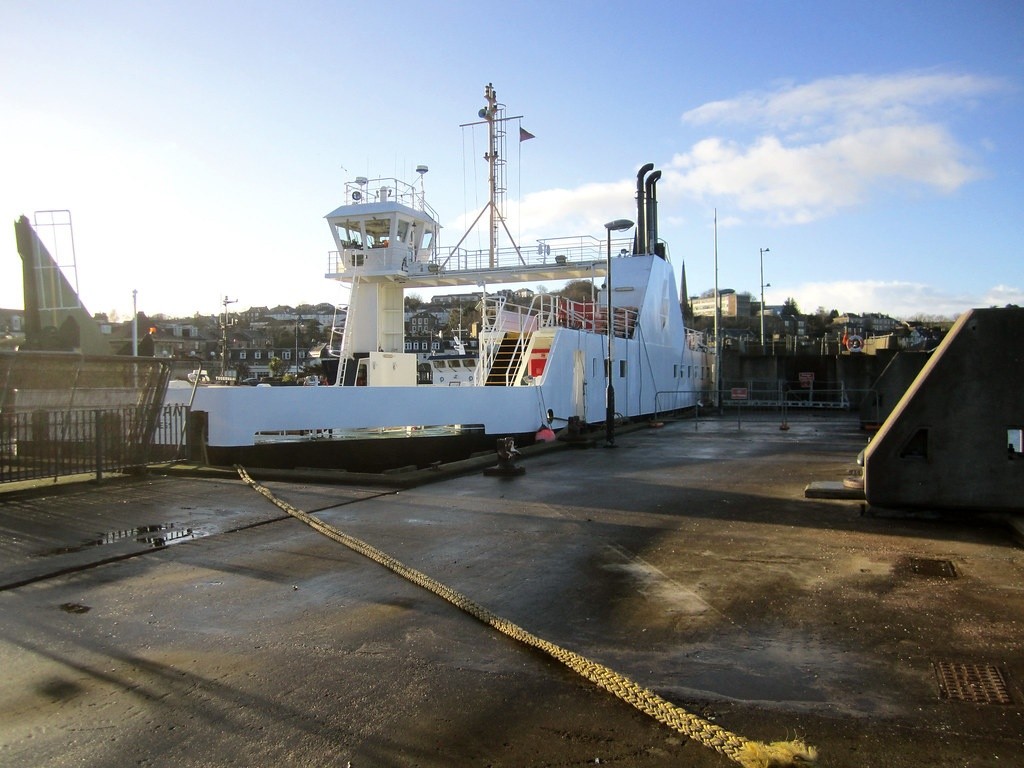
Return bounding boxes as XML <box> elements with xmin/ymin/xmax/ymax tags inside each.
<box><xmin>242</xmin><ymin>377</ymin><xmax>259</xmax><ymax>385</ymax></box>
<box><xmin>256</xmin><ymin>384</ymin><xmax>271</xmax><ymax>387</ymax></box>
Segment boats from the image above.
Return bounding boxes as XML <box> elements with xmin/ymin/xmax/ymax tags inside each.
<box><xmin>129</xmin><ymin>81</ymin><xmax>729</xmax><ymax>473</ymax></box>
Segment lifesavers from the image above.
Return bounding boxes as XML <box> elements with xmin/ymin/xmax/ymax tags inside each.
<box><xmin>846</xmin><ymin>335</ymin><xmax>865</xmax><ymax>352</ymax></box>
<box><xmin>352</xmin><ymin>192</ymin><xmax>360</xmax><ymax>200</ymax></box>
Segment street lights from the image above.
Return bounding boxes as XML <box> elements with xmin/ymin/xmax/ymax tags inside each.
<box><xmin>760</xmin><ymin>246</ymin><xmax>770</xmax><ymax>355</ymax></box>
<box><xmin>603</xmin><ymin>219</ymin><xmax>635</xmax><ymax>449</ymax></box>
